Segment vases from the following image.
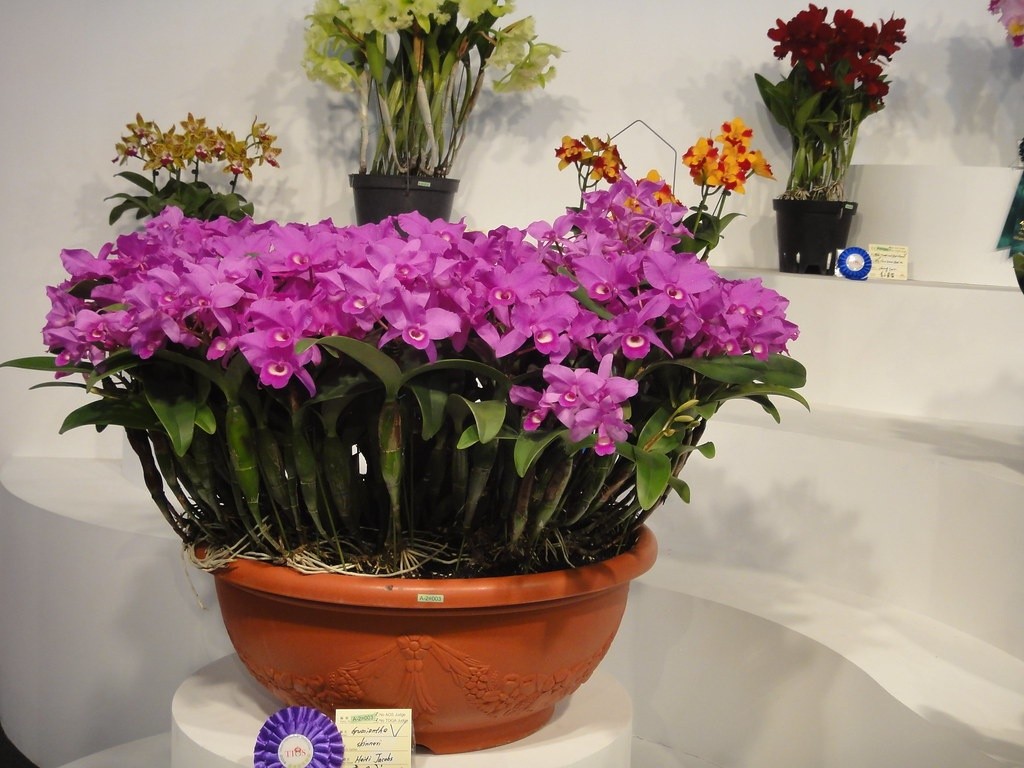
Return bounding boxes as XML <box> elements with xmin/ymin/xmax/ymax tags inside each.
<box><xmin>347</xmin><ymin>173</ymin><xmax>461</xmax><ymax>226</ymax></box>
<box><xmin>773</xmin><ymin>197</ymin><xmax>858</xmax><ymax>277</ymax></box>
<box><xmin>185</xmin><ymin>526</ymin><xmax>659</xmax><ymax>757</ymax></box>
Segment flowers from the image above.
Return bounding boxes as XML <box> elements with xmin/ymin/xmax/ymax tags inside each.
<box><xmin>0</xmin><ymin>0</ymin><xmax>1024</xmax><ymax>581</ymax></box>
